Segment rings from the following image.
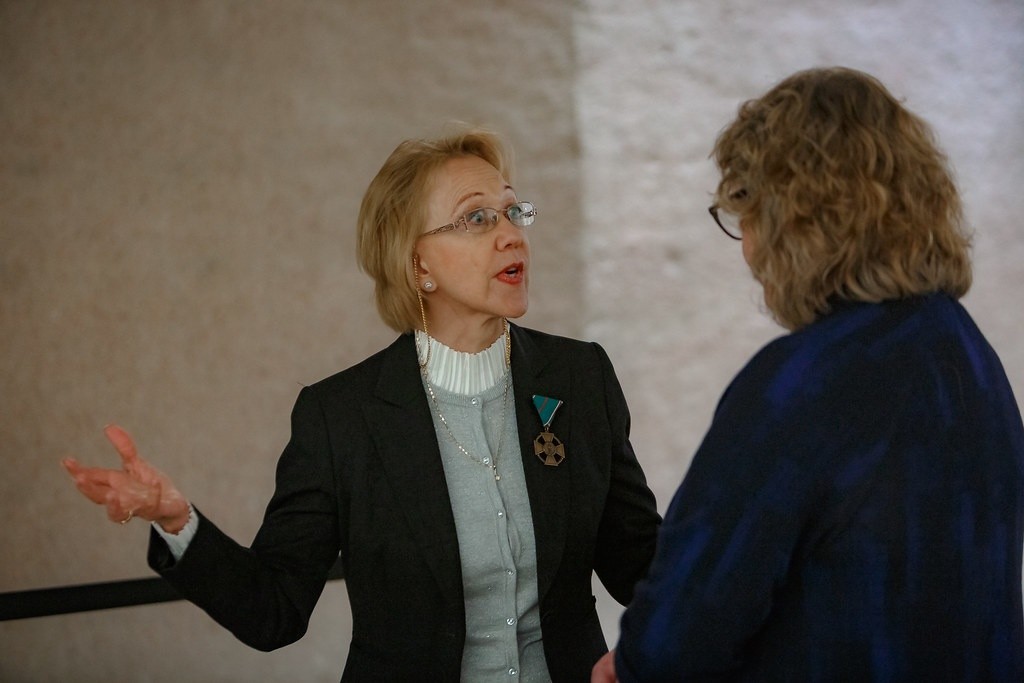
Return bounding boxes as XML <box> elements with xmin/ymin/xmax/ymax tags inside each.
<box><xmin>121</xmin><ymin>510</ymin><xmax>133</xmax><ymax>524</ymax></box>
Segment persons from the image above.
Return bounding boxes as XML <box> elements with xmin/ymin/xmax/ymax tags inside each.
<box><xmin>587</xmin><ymin>65</ymin><xmax>1024</xmax><ymax>683</ymax></box>
<box><xmin>62</xmin><ymin>118</ymin><xmax>663</xmax><ymax>683</ymax></box>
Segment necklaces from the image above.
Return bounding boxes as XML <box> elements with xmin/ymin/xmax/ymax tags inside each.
<box><xmin>416</xmin><ymin>317</ymin><xmax>512</xmax><ymax>479</ymax></box>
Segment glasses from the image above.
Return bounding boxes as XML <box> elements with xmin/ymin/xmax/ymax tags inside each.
<box><xmin>709</xmin><ymin>187</ymin><xmax>747</xmax><ymax>240</ymax></box>
<box><xmin>420</xmin><ymin>201</ymin><xmax>537</xmax><ymax>237</ymax></box>
